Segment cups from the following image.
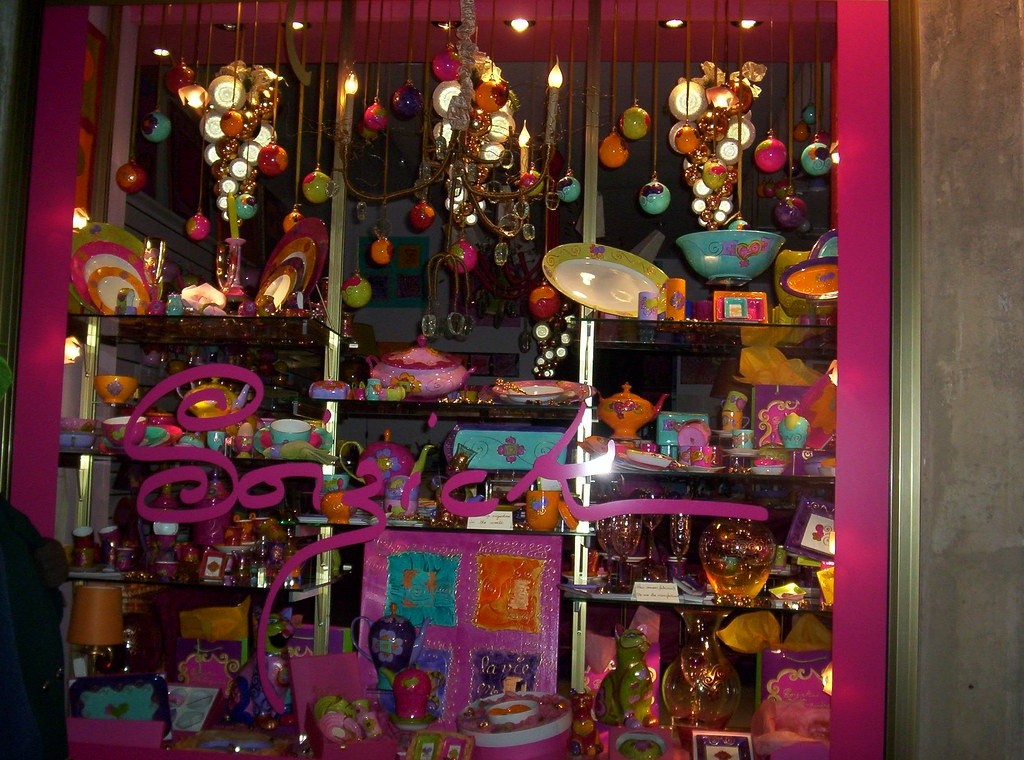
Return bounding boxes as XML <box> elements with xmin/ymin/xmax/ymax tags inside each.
<box><xmin>721</xmin><ymin>410</ymin><xmax>750</xmax><ymax>429</ymax></box>
<box><xmin>353</xmin><ymin>699</ymin><xmax>381</xmax><ymax>739</ymax></box>
<box><xmin>117</xmin><ymin>548</ymin><xmax>134</xmax><ymax>570</ymax></box>
<box><xmin>236</xmin><ymin>517</ymin><xmax>270</xmax><ymax>545</ymax></box>
<box><xmin>526</xmin><ymin>491</ymin><xmax>561</xmax><ymax>532</ymax></box>
<box><xmin>731</xmin><ymin>430</ymin><xmax>754</xmax><ymax>449</ymax></box>
<box><xmin>337</xmin><ymin>439</ymin><xmax>349</xmax><ymax>457</ymax></box>
<box><xmin>675</xmin><ymin>419</ymin><xmax>712</xmax><ymax>446</ymax></box>
<box><xmin>681</xmin><ymin>445</ymin><xmax>716</xmax><ymax>467</ymax></box>
<box><xmin>157</xmin><ymin>562</ymin><xmax>178</xmax><ymax>581</ymax></box>
<box><xmin>721</xmin><ymin>391</ymin><xmax>749</xmax><ymax>411</ymax></box>
<box><xmin>99</xmin><ymin>526</ymin><xmax>120</xmax><ymax>572</ymax></box>
<box><xmin>446</xmin><ymin>443</ymin><xmax>478</xmax><ymax>478</ymax></box>
<box><xmin>72</xmin><ymin>526</ymin><xmax>96</xmax><ymax>572</ymax></box>
<box><xmin>207</xmin><ymin>431</ymin><xmax>225</xmax><ymax>451</ymax></box>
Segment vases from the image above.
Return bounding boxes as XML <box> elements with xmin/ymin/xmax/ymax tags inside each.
<box><xmin>662</xmin><ymin>606</ymin><xmax>741</xmax><ymax>731</ymax></box>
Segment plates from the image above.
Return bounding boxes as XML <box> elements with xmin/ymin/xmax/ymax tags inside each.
<box><xmin>88</xmin><ymin>268</ymin><xmax>148</xmax><ymax>315</ymax></box>
<box><xmin>255</xmin><ymin>264</ymin><xmax>297</xmax><ymax>314</ymax></box>
<box><xmin>215</xmin><ymin>545</ymin><xmax>251</xmax><ymax>553</ymax></box>
<box><xmin>258</xmin><ymin>237</ymin><xmax>315</xmax><ymax>313</ymax></box>
<box><xmin>70</xmin><ymin>222</ymin><xmax>158</xmax><ymax>315</ymax></box>
<box><xmin>70</xmin><ymin>241</ymin><xmax>153</xmax><ymax>315</ymax></box>
<box><xmin>562</xmin><ymin>570</ymin><xmax>608</xmax><ymax>579</ymax></box>
<box><xmin>722</xmin><ymin>449</ymin><xmax>759</xmax><ymax>456</ymax></box>
<box><xmin>779</xmin><ymin>256</ymin><xmax>839</xmax><ymax>299</ymax></box>
<box><xmin>314</xmin><ymin>696</ymin><xmax>361</xmax><ymax>746</ymax></box>
<box><xmin>104</xmin><ymin>426</ymin><xmax>171</xmax><ymax>447</ymax></box>
<box><xmin>260</xmin><ymin>217</ymin><xmax>329</xmax><ymax>313</ymax></box>
<box><xmin>809</xmin><ymin>229</ymin><xmax>838</xmax><ymax>258</ymax></box>
<box><xmin>681</xmin><ymin>465</ymin><xmax>726</xmax><ymax>473</ymax></box>
<box><xmin>492</xmin><ymin>380</ymin><xmax>577</xmax><ymax>405</ymax></box>
<box><xmin>749</xmin><ymin>465</ymin><xmax>785</xmax><ymax>475</ymax></box>
<box><xmin>712</xmin><ymin>430</ymin><xmax>733</xmax><ymax>438</ymax></box>
<box><xmin>253</xmin><ymin>424</ymin><xmax>333</xmax><ymax>455</ymax></box>
<box><xmin>542</xmin><ymin>243</ymin><xmax>671</xmax><ymax>318</ymax></box>
<box><xmin>585</xmin><ymin>435</ymin><xmax>679</xmax><ymax>471</ymax></box>
<box><xmin>386</xmin><ymin>519</ymin><xmax>417</xmax><ymax>526</ymax></box>
<box><xmin>99</xmin><ymin>425</ymin><xmax>183</xmax><ymax>452</ymax></box>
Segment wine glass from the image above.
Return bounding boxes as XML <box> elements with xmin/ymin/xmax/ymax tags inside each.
<box><xmin>641</xmin><ymin>493</ymin><xmax>669</xmax><ymax>583</ymax></box>
<box><xmin>597</xmin><ymin>502</ymin><xmax>642</xmax><ymax>594</ymax></box>
<box><xmin>666</xmin><ymin>494</ymin><xmax>692</xmax><ymax>581</ymax></box>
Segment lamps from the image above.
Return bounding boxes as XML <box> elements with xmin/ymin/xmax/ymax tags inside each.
<box><xmin>333</xmin><ymin>1</ymin><xmax>570</xmax><ymax>337</ymax></box>
<box><xmin>110</xmin><ymin>2</ymin><xmax>306</xmax><ymax>234</ymax></box>
<box><xmin>65</xmin><ymin>585</ymin><xmax>125</xmax><ymax>675</ymax></box>
<box><xmin>504</xmin><ymin>17</ymin><xmax>536</xmax><ymax>34</ymax></box>
<box><xmin>27</xmin><ymin>0</ymin><xmax>892</xmax><ymax>760</ymax></box>
<box><xmin>664</xmin><ymin>0</ymin><xmax>766</xmax><ymax>231</ymax></box>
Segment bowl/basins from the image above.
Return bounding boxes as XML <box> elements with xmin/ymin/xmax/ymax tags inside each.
<box><xmin>94</xmin><ymin>375</ymin><xmax>137</xmax><ymax>404</ymax></box>
<box><xmin>59</xmin><ymin>433</ymin><xmax>94</xmax><ymax>448</ymax></box>
<box><xmin>60</xmin><ymin>419</ymin><xmax>98</xmax><ymax>431</ymax></box>
<box><xmin>270</xmin><ymin>419</ymin><xmax>312</xmax><ymax>446</ymax></box>
<box><xmin>676</xmin><ymin>230</ymin><xmax>786</xmax><ymax>280</ymax></box>
<box><xmin>322</xmin><ymin>474</ymin><xmax>349</xmax><ymax>491</ymax></box>
<box><xmin>103</xmin><ymin>416</ymin><xmax>147</xmax><ymax>447</ymax></box>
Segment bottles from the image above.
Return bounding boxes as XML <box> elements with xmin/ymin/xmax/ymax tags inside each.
<box><xmin>232</xmin><ymin>535</ymin><xmax>302</xmax><ymax>591</ymax></box>
<box><xmin>661</xmin><ymin>605</ymin><xmax>743</xmax><ymax>730</ymax></box>
<box><xmin>699</xmin><ymin>508</ymin><xmax>773</xmax><ymax>601</ymax></box>
<box><xmin>778</xmin><ymin>412</ymin><xmax>811</xmax><ymax>448</ymax></box>
<box><xmin>194</xmin><ymin>462</ymin><xmax>230</xmax><ymax>546</ymax></box>
<box><xmin>154</xmin><ymin>470</ymin><xmax>178</xmax><ymax>551</ymax></box>
<box><xmin>638</xmin><ymin>291</ymin><xmax>659</xmax><ymax>320</ymax></box>
<box><xmin>666</xmin><ymin>278</ymin><xmax>686</xmax><ymax>321</ymax></box>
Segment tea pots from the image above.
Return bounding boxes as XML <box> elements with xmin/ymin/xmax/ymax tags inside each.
<box><xmin>350</xmin><ymin>603</ymin><xmax>430</xmax><ymax>690</ymax></box>
<box><xmin>556</xmin><ymin>381</ymin><xmax>671</xmax><ymax>439</ymax></box>
<box><xmin>321</xmin><ymin>477</ymin><xmax>360</xmax><ymax>524</ymax></box>
<box><xmin>339</xmin><ymin>428</ymin><xmax>434</xmax><ymax>495</ymax></box>
<box><xmin>367</xmin><ymin>336</ymin><xmax>477</xmax><ymax>402</ymax></box>
<box><xmin>140</xmin><ymin>347</ymin><xmax>161</xmax><ymax>365</ymax></box>
<box><xmin>183</xmin><ymin>375</ymin><xmax>252</xmax><ymax>422</ymax></box>
<box><xmin>381</xmin><ymin>663</ymin><xmax>446</xmax><ymax>730</ymax></box>
<box><xmin>309</xmin><ymin>378</ymin><xmax>350</xmax><ymax>399</ymax></box>
